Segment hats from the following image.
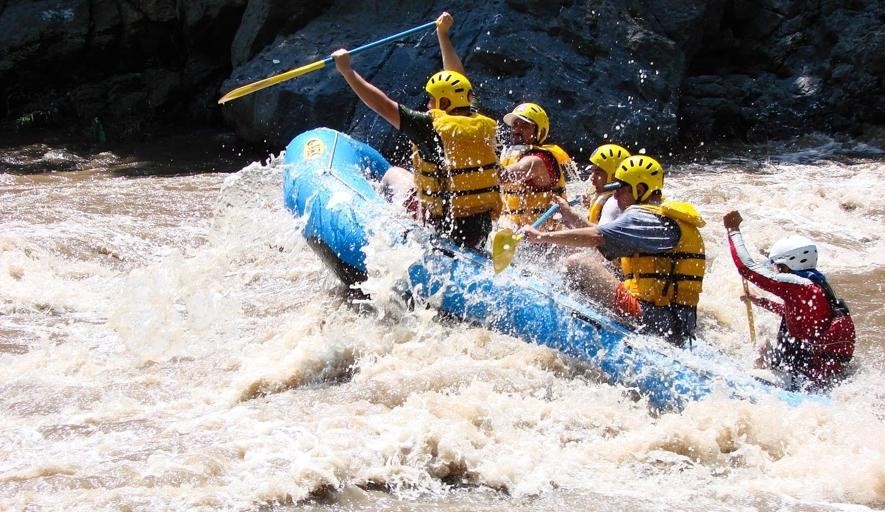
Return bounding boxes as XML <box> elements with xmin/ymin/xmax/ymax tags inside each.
<box><xmin>503</xmin><ymin>113</ymin><xmax>535</xmax><ymax>126</ymax></box>
<box><xmin>603</xmin><ymin>179</ymin><xmax>625</xmax><ymax>190</ymax></box>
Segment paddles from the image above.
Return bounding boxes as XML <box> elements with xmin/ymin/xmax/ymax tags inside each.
<box><xmin>493</xmin><ymin>204</ymin><xmax>559</xmax><ymax>275</ymax></box>
<box><xmin>218</xmin><ymin>19</ymin><xmax>442</xmax><ymax>104</ymax></box>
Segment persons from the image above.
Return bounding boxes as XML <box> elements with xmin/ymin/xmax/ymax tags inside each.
<box><xmin>331</xmin><ymin>12</ymin><xmax>503</xmax><ymax>249</ymax></box>
<box><xmin>576</xmin><ymin>143</ymin><xmax>632</xmax><ymax>224</ymax></box>
<box><xmin>722</xmin><ymin>210</ymin><xmax>855</xmax><ymax>393</ymax></box>
<box><xmin>497</xmin><ymin>103</ymin><xmax>572</xmax><ymax>247</ymax></box>
<box><xmin>519</xmin><ymin>155</ymin><xmax>706</xmax><ymax>348</ymax></box>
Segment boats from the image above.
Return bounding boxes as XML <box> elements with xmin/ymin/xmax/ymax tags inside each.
<box><xmin>277</xmin><ymin>122</ymin><xmax>879</xmax><ymax>452</ymax></box>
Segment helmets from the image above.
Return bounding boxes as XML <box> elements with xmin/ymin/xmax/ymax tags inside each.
<box><xmin>425</xmin><ymin>71</ymin><xmax>473</xmax><ymax>113</ymax></box>
<box><xmin>512</xmin><ymin>103</ymin><xmax>550</xmax><ymax>144</ymax></box>
<box><xmin>589</xmin><ymin>144</ymin><xmax>664</xmax><ymax>201</ymax></box>
<box><xmin>769</xmin><ymin>235</ymin><xmax>818</xmax><ymax>271</ymax></box>
